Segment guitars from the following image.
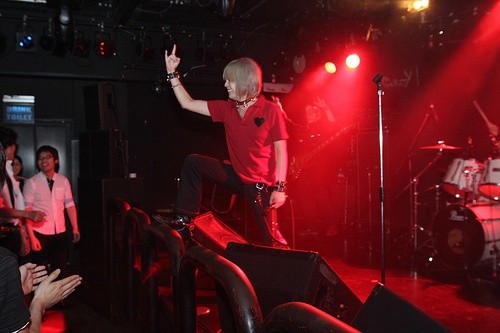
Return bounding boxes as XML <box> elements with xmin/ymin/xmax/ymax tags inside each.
<box><xmin>287</xmin><ymin>123</ymin><xmax>352</xmax><ymax>182</ymax></box>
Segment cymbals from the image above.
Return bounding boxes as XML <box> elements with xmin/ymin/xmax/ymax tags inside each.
<box><xmin>419</xmin><ymin>144</ymin><xmax>463</xmax><ymax>150</ymax></box>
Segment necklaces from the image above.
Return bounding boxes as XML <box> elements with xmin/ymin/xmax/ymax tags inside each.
<box><xmin>235</xmin><ymin>96</ymin><xmax>257</xmax><ymax>107</ymax></box>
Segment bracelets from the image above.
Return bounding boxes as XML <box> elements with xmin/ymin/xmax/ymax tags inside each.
<box><xmin>274</xmin><ymin>185</ymin><xmax>285</xmax><ymax>192</ymax></box>
<box><xmin>275</xmin><ymin>181</ymin><xmax>287</xmax><ymax>187</ymax></box>
<box><xmin>167</xmin><ymin>72</ymin><xmax>180</xmax><ymax>81</ymax></box>
<box><xmin>171</xmin><ymin>83</ymin><xmax>183</xmax><ymax>88</ymax></box>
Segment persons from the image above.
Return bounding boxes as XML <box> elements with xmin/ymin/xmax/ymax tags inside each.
<box><xmin>151</xmin><ymin>44</ymin><xmax>289</xmax><ymax>250</ymax></box>
<box><xmin>0</xmin><ymin>245</ymin><xmax>82</xmax><ymax>333</ymax></box>
<box><xmin>19</xmin><ymin>145</ymin><xmax>81</xmax><ymax>266</ymax></box>
<box><xmin>0</xmin><ymin>125</ymin><xmax>46</xmax><ymax>260</ymax></box>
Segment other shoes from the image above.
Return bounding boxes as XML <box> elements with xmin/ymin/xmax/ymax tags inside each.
<box><xmin>152</xmin><ymin>207</ymin><xmax>190</xmax><ymax>233</ymax></box>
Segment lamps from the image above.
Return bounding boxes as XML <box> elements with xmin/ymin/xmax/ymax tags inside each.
<box><xmin>395</xmin><ymin>0</ymin><xmax>480</xmax><ymax>52</ymax></box>
<box><xmin>16</xmin><ymin>0</ymin><xmax>236</xmax><ymax>62</ymax></box>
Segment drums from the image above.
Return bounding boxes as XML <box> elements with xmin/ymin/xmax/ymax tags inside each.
<box><xmin>431</xmin><ymin>200</ymin><xmax>500</xmax><ymax>272</ymax></box>
<box><xmin>477</xmin><ymin>156</ymin><xmax>500</xmax><ymax>202</ymax></box>
<box><xmin>439</xmin><ymin>158</ymin><xmax>486</xmax><ymax>201</ymax></box>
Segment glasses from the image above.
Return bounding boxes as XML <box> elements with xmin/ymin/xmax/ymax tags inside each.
<box><xmin>37</xmin><ymin>154</ymin><xmax>54</xmax><ymax>162</ymax></box>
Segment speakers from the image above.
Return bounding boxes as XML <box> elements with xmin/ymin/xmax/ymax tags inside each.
<box><xmin>74</xmin><ymin>83</ymin><xmax>180</xmax><ymax>323</ymax></box>
<box><xmin>189</xmin><ymin>210</ymin><xmax>452</xmax><ymax>333</ymax></box>
